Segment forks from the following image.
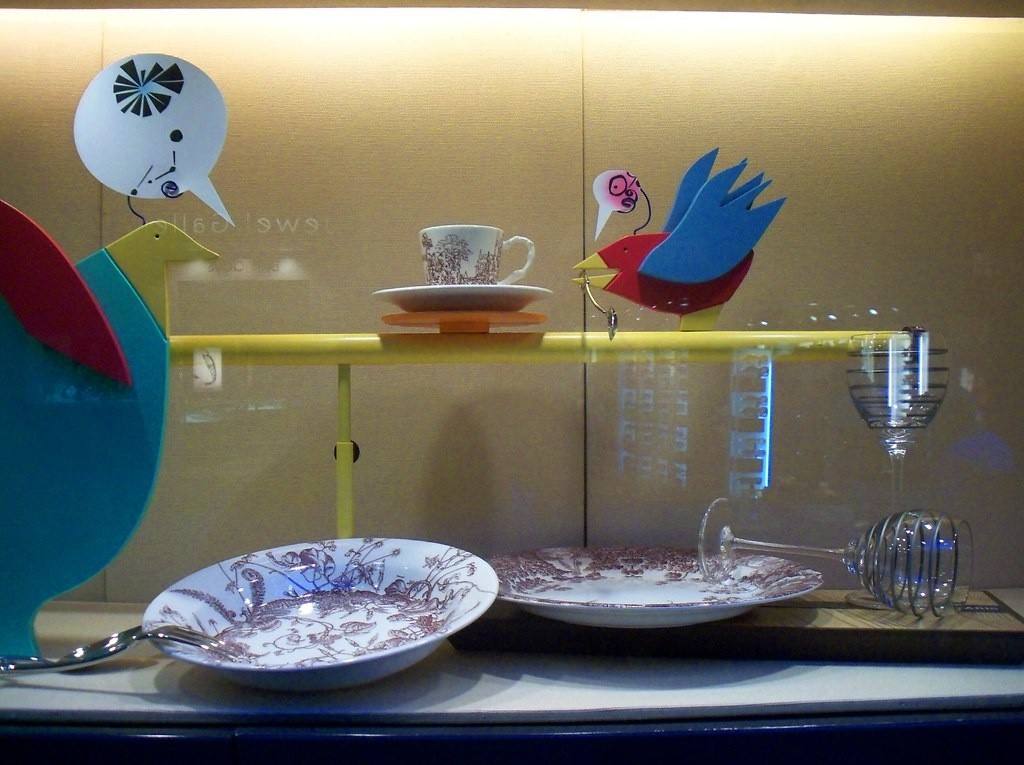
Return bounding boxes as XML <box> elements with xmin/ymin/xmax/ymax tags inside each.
<box><xmin>136</xmin><ymin>626</ymin><xmax>244</xmax><ymax>673</ymax></box>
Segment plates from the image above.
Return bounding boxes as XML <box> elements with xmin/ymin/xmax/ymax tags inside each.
<box><xmin>370</xmin><ymin>285</ymin><xmax>553</xmax><ymax>312</ymax></box>
<box><xmin>141</xmin><ymin>536</ymin><xmax>500</xmax><ymax>690</ymax></box>
<box><xmin>477</xmin><ymin>545</ymin><xmax>824</xmax><ymax>629</ymax></box>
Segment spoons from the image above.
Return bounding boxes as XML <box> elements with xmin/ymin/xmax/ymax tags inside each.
<box><xmin>0</xmin><ymin>625</ymin><xmax>140</xmax><ymax>670</ymax></box>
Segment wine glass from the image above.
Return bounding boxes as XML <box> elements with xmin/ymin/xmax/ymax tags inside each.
<box><xmin>698</xmin><ymin>498</ymin><xmax>975</xmax><ymax>617</ymax></box>
<box><xmin>846</xmin><ymin>329</ymin><xmax>952</xmax><ymax>610</ymax></box>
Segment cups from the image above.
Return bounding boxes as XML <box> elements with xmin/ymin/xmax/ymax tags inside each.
<box><xmin>419</xmin><ymin>224</ymin><xmax>535</xmax><ymax>285</ymax></box>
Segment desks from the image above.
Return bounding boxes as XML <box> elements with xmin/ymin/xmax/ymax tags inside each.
<box><xmin>0</xmin><ymin>609</ymin><xmax>1023</xmax><ymax>765</ymax></box>
